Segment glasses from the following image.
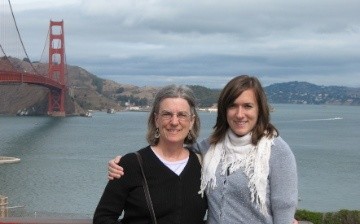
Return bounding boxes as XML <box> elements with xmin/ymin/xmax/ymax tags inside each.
<box><xmin>160</xmin><ymin>112</ymin><xmax>192</xmax><ymax>120</ymax></box>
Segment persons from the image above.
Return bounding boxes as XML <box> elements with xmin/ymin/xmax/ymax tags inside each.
<box><xmin>108</xmin><ymin>75</ymin><xmax>298</xmax><ymax>224</ymax></box>
<box><xmin>92</xmin><ymin>84</ymin><xmax>298</xmax><ymax>224</ymax></box>
